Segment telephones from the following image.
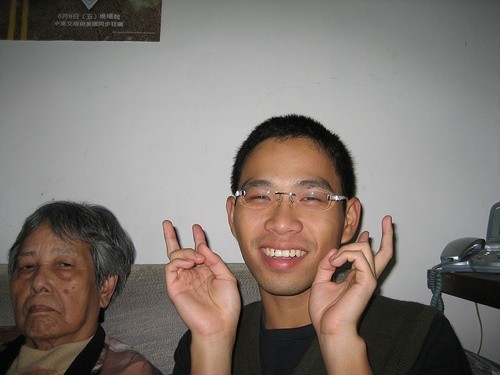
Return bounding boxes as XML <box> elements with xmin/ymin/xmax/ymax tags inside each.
<box><xmin>440</xmin><ymin>201</ymin><xmax>500</xmax><ymax>273</ymax></box>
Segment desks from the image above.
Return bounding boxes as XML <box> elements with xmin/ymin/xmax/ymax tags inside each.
<box><xmin>427</xmin><ymin>269</ymin><xmax>500</xmax><ymax>310</ymax></box>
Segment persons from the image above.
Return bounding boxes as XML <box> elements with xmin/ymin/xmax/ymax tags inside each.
<box><xmin>162</xmin><ymin>113</ymin><xmax>475</xmax><ymax>375</ymax></box>
<box><xmin>1</xmin><ymin>199</ymin><xmax>162</xmax><ymax>375</ymax></box>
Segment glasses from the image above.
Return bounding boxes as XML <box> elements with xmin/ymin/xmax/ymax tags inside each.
<box><xmin>235</xmin><ymin>184</ymin><xmax>349</xmax><ymax>212</ymax></box>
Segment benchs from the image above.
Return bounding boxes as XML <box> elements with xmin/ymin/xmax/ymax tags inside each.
<box><xmin>0</xmin><ymin>263</ymin><xmax>500</xmax><ymax>375</ymax></box>
